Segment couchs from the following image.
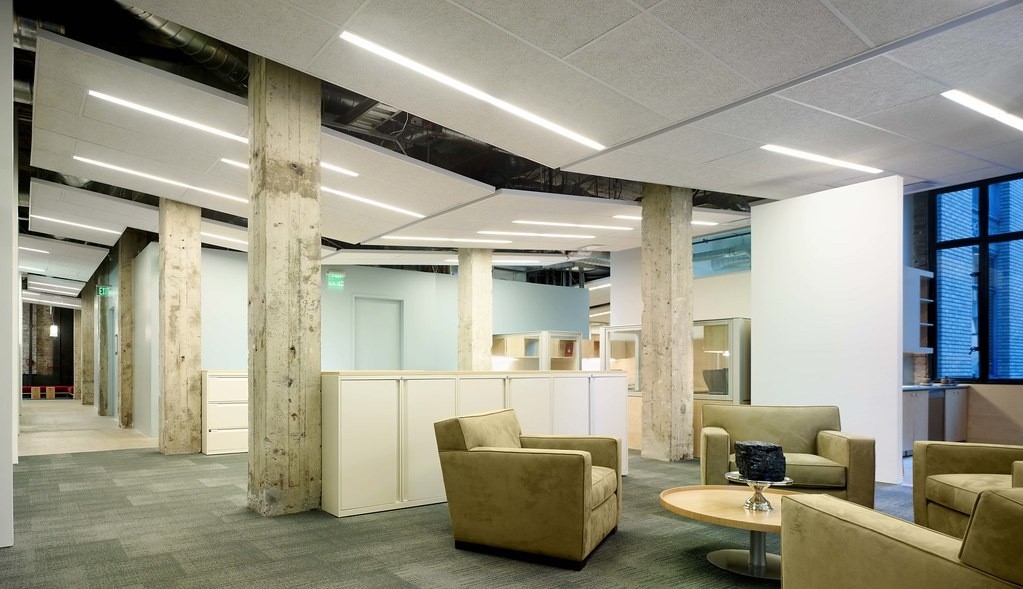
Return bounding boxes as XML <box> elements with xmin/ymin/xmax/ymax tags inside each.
<box><xmin>913</xmin><ymin>441</ymin><xmax>1023</xmax><ymax>538</ymax></box>
<box><xmin>781</xmin><ymin>488</ymin><xmax>1023</xmax><ymax>589</ymax></box>
<box><xmin>433</xmin><ymin>408</ymin><xmax>622</xmax><ymax>571</ymax></box>
<box><xmin>701</xmin><ymin>404</ymin><xmax>875</xmax><ymax>509</ymax></box>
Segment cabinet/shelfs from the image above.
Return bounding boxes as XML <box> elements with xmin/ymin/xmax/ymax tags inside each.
<box><xmin>903</xmin><ymin>266</ymin><xmax>933</xmax><ymax>353</ymax></box>
<box><xmin>201</xmin><ymin>370</ymin><xmax>250</xmax><ymax>455</ymax></box>
<box><xmin>903</xmin><ymin>388</ymin><xmax>969</xmax><ymax>457</ymax></box>
<box><xmin>322</xmin><ymin>371</ymin><xmax>628</xmax><ymax>518</ymax></box>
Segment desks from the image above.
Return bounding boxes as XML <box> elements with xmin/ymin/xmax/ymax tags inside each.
<box><xmin>660</xmin><ymin>485</ymin><xmax>805</xmax><ymax>579</ymax></box>
